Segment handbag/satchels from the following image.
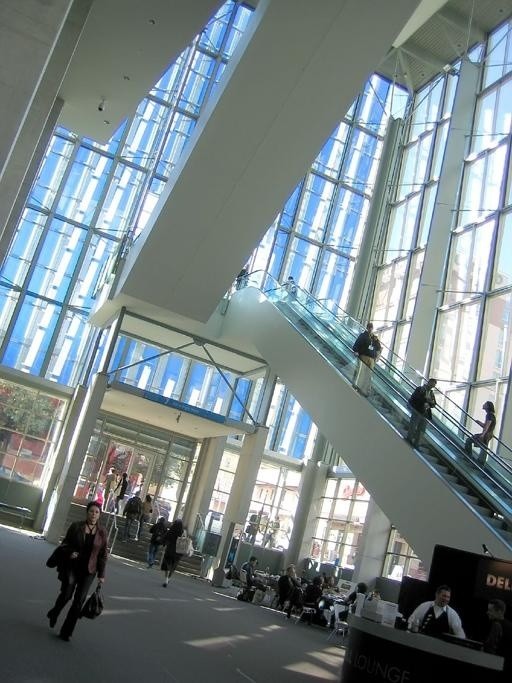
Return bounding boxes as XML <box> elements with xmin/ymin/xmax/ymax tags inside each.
<box><xmin>80</xmin><ymin>581</ymin><xmax>105</xmax><ymax>621</ymax></box>
<box><xmin>175</xmin><ymin>529</ymin><xmax>193</xmax><ymax>555</ymax></box>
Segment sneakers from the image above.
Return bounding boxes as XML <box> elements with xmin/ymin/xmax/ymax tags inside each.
<box><xmin>47</xmin><ymin>611</ymin><xmax>71</xmax><ymax>643</ymax></box>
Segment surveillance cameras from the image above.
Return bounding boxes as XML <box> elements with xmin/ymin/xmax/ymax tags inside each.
<box><xmin>98</xmin><ymin>99</ymin><xmax>105</xmax><ymax>111</ymax></box>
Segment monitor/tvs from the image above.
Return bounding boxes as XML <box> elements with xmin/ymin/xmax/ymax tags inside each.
<box><xmin>441</xmin><ymin>631</ymin><xmax>484</xmax><ymax>653</ymax></box>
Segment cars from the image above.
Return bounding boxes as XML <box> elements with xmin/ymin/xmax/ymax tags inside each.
<box><xmin>83</xmin><ymin>480</ymin><xmax>290</xmax><ymax>551</ymax></box>
<box><xmin>1</xmin><ymin>466</ymin><xmax>38</xmax><ymax>487</ymax></box>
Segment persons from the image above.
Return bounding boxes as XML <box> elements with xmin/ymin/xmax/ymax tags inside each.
<box><xmin>145</xmin><ymin>517</ymin><xmax>168</xmax><ymax>570</ymax></box>
<box><xmin>406</xmin><ymin>377</ymin><xmax>438</xmax><ymax>453</ymax></box>
<box><xmin>351</xmin><ymin>322</ymin><xmax>382</xmax><ymax>396</ymax></box>
<box><xmin>261</xmin><ymin>514</ymin><xmax>282</xmax><ymax>549</ymax></box>
<box><xmin>480</xmin><ymin>599</ymin><xmax>508</xmax><ymax>657</ymax></box>
<box><xmin>237</xmin><ymin>555</ymin><xmax>369</xmax><ymax>636</ymax></box>
<box><xmin>134</xmin><ymin>494</ymin><xmax>153</xmax><ymax>543</ymax></box>
<box><xmin>461</xmin><ymin>401</ymin><xmax>497</xmax><ymax>472</ymax></box>
<box><xmin>406</xmin><ymin>583</ymin><xmax>467</xmax><ymax>641</ymax></box>
<box><xmin>46</xmin><ymin>501</ymin><xmax>108</xmax><ymax>640</ymax></box>
<box><xmin>122</xmin><ymin>491</ymin><xmax>143</xmax><ymax>543</ymax></box>
<box><xmin>102</xmin><ymin>467</ymin><xmax>132</xmax><ymax>515</ymax></box>
<box><xmin>283</xmin><ymin>280</ymin><xmax>298</xmax><ymax>302</ymax></box>
<box><xmin>158</xmin><ymin>518</ymin><xmax>187</xmax><ymax>588</ymax></box>
<box><xmin>281</xmin><ymin>275</ymin><xmax>294</xmax><ymax>292</ymax></box>
<box><xmin>236</xmin><ymin>263</ymin><xmax>251</xmax><ymax>289</ymax></box>
<box><xmin>244</xmin><ymin>509</ymin><xmax>263</xmax><ymax>546</ymax></box>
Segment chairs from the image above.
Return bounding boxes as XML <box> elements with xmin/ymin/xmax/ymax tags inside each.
<box><xmin>238</xmin><ymin>560</ymin><xmax>368</xmax><ymax>644</ymax></box>
